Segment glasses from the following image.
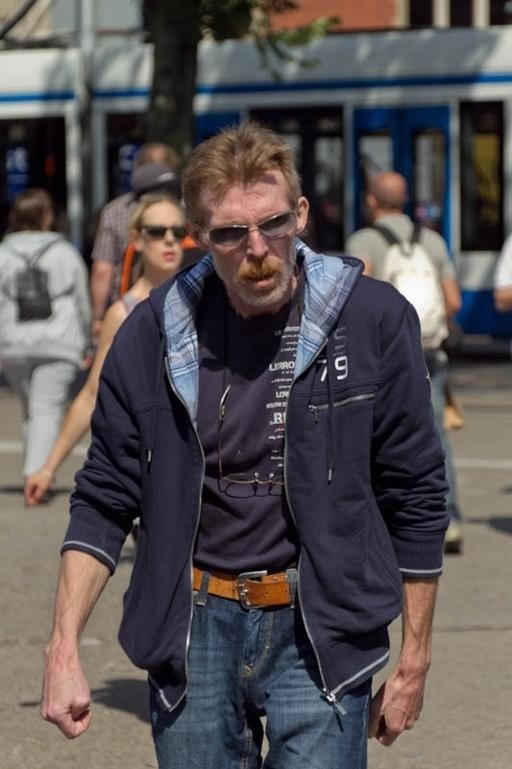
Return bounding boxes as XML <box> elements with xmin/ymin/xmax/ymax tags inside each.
<box><xmin>137</xmin><ymin>224</ymin><xmax>187</xmax><ymax>240</ymax></box>
<box><xmin>216</xmin><ymin>384</ymin><xmax>284</xmax><ymax>486</ymax></box>
<box><xmin>199</xmin><ymin>211</ymin><xmax>298</xmax><ymax>249</ymax></box>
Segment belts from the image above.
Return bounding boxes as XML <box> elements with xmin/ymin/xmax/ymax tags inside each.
<box><xmin>189</xmin><ymin>566</ymin><xmax>290</xmax><ymax>610</ymax></box>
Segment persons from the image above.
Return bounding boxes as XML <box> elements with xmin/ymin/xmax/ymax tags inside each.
<box><xmin>1</xmin><ymin>185</ymin><xmax>97</xmax><ymax>495</ymax></box>
<box><xmin>87</xmin><ymin>138</ymin><xmax>181</xmax><ymax>324</ymax></box>
<box><xmin>441</xmin><ymin>316</ymin><xmax>461</xmax><ymax>431</ymax></box>
<box><xmin>344</xmin><ymin>167</ymin><xmax>464</xmax><ymax>441</ymax></box>
<box><xmin>23</xmin><ymin>192</ymin><xmax>183</xmax><ymax>506</ymax></box>
<box><xmin>41</xmin><ymin>122</ymin><xmax>447</xmax><ymax>768</ymax></box>
<box><xmin>492</xmin><ymin>223</ymin><xmax>510</xmax><ymax>322</ymax></box>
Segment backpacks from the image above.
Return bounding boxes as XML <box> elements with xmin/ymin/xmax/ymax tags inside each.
<box><xmin>370</xmin><ymin>226</ymin><xmax>451</xmax><ymax>350</ymax></box>
<box><xmin>0</xmin><ymin>240</ymin><xmax>76</xmax><ymax>323</ymax></box>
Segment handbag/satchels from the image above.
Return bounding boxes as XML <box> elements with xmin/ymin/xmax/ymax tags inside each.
<box><xmin>442</xmin><ymin>378</ymin><xmax>463</xmax><ymax>431</ymax></box>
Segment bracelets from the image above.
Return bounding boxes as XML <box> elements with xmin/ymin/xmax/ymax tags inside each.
<box><xmin>39</xmin><ymin>467</ymin><xmax>56</xmax><ymax>482</ymax></box>
